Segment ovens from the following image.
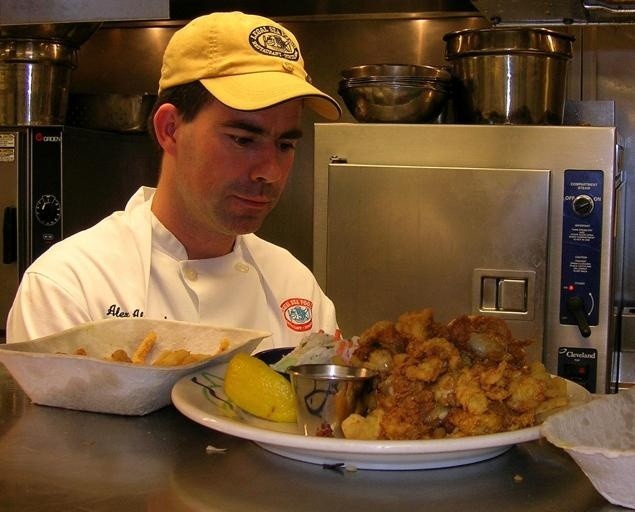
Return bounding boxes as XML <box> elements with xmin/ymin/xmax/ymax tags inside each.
<box><xmin>0</xmin><ymin>126</ymin><xmax>154</xmax><ymax>333</ymax></box>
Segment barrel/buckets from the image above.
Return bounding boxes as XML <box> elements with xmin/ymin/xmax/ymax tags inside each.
<box><xmin>0</xmin><ymin>39</ymin><xmax>19</xmax><ymax>127</ymax></box>
<box><xmin>11</xmin><ymin>36</ymin><xmax>76</xmax><ymax>127</ymax></box>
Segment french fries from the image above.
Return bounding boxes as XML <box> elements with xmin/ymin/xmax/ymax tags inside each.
<box><xmin>53</xmin><ymin>330</ymin><xmax>231</xmax><ymax>366</ymax></box>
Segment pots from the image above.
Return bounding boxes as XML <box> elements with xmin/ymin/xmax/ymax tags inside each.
<box><xmin>0</xmin><ymin>33</ymin><xmax>82</xmax><ymax>130</ymax></box>
<box><xmin>442</xmin><ymin>16</ymin><xmax>577</xmax><ymax>127</ymax></box>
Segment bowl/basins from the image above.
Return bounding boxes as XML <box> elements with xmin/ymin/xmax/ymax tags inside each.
<box><xmin>69</xmin><ymin>86</ymin><xmax>159</xmax><ymax>135</ymax></box>
<box><xmin>537</xmin><ymin>383</ymin><xmax>635</xmax><ymax>510</ymax></box>
<box><xmin>334</xmin><ymin>52</ymin><xmax>453</xmax><ymax>122</ymax></box>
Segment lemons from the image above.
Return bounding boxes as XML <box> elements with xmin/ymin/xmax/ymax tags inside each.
<box><xmin>222</xmin><ymin>351</ymin><xmax>297</xmax><ymax>422</ymax></box>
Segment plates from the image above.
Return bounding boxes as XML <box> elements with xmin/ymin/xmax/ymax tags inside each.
<box><xmin>168</xmin><ymin>358</ymin><xmax>595</xmax><ymax>473</ymax></box>
<box><xmin>1</xmin><ymin>314</ymin><xmax>274</xmax><ymax>418</ymax></box>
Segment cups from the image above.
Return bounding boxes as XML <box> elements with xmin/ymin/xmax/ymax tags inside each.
<box><xmin>283</xmin><ymin>364</ymin><xmax>380</xmax><ymax>436</ymax></box>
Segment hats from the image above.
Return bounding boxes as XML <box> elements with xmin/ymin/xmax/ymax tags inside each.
<box><xmin>158</xmin><ymin>11</ymin><xmax>343</xmax><ymax>122</ymax></box>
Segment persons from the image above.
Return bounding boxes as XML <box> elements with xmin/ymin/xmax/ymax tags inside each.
<box><xmin>4</xmin><ymin>10</ymin><xmax>347</xmax><ymax>355</ymax></box>
